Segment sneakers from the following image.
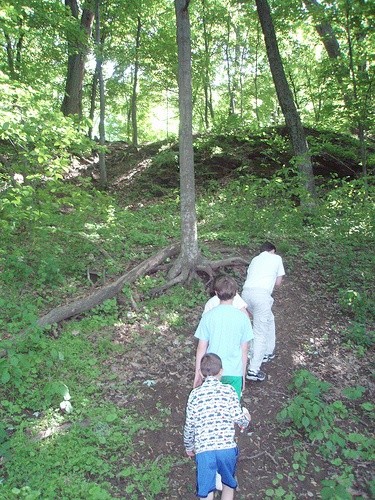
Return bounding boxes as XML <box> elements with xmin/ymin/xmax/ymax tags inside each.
<box><xmin>263</xmin><ymin>354</ymin><xmax>274</xmax><ymax>362</ymax></box>
<box><xmin>246</xmin><ymin>370</ymin><xmax>265</xmax><ymax>381</ymax></box>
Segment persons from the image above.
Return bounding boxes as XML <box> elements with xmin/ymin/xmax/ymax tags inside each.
<box><xmin>240</xmin><ymin>240</ymin><xmax>287</xmax><ymax>381</ymax></box>
<box><xmin>202</xmin><ymin>276</ymin><xmax>248</xmax><ymax>320</ymax></box>
<box><xmin>192</xmin><ymin>275</ymin><xmax>255</xmax><ymax>491</ymax></box>
<box><xmin>183</xmin><ymin>352</ymin><xmax>251</xmax><ymax>500</ymax></box>
